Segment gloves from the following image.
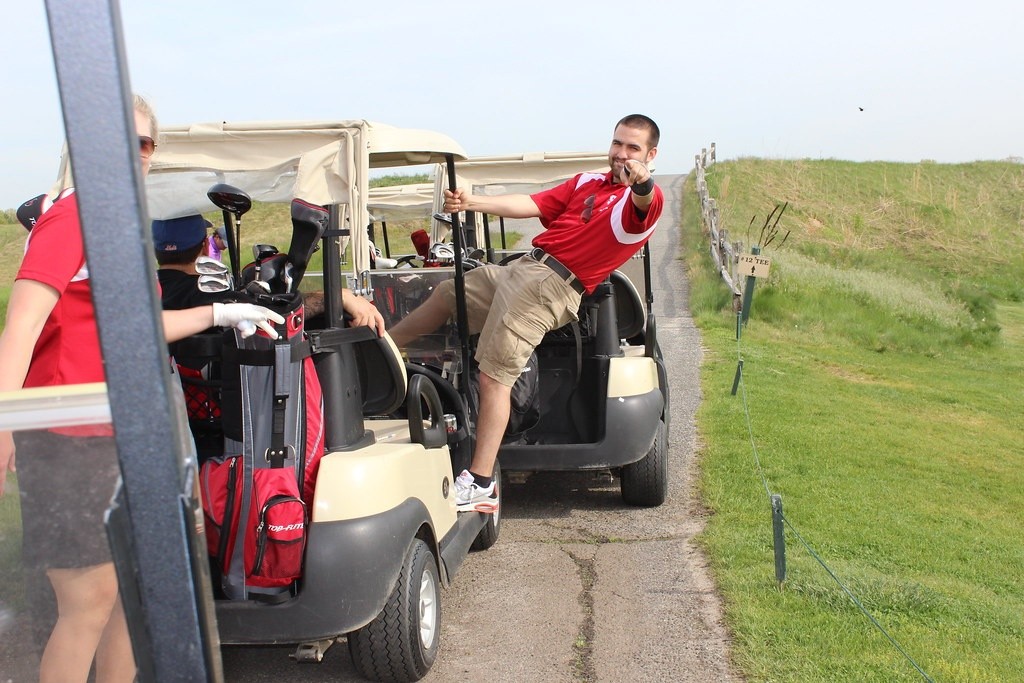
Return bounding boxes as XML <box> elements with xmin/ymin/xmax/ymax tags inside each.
<box><xmin>212</xmin><ymin>303</ymin><xmax>286</xmax><ymax>339</ymax></box>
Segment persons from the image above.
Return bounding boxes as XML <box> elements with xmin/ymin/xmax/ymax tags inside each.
<box><xmin>153</xmin><ymin>213</ymin><xmax>397</xmax><ymax>375</ymax></box>
<box><xmin>387</xmin><ymin>114</ymin><xmax>664</xmax><ymax>513</ymax></box>
<box><xmin>0</xmin><ymin>93</ymin><xmax>286</xmax><ymax>682</ymax></box>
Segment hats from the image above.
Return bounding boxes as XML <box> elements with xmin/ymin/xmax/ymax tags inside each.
<box><xmin>216</xmin><ymin>227</ymin><xmax>229</xmax><ymax>248</ymax></box>
<box><xmin>151</xmin><ymin>214</ymin><xmax>213</xmax><ymax>253</ymax></box>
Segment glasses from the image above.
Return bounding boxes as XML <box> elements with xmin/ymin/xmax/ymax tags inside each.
<box><xmin>137</xmin><ymin>136</ymin><xmax>156</xmax><ymax>159</ymax></box>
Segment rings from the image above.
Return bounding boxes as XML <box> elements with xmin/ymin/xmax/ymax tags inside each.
<box><xmin>443</xmin><ymin>203</ymin><xmax>446</xmax><ymax>208</ymax></box>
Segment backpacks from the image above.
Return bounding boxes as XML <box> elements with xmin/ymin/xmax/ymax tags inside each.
<box><xmin>200</xmin><ymin>456</ymin><xmax>305</xmax><ymax>587</ymax></box>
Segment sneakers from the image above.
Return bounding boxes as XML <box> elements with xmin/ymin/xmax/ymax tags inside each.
<box><xmin>454</xmin><ymin>469</ymin><xmax>500</xmax><ymax>513</ymax></box>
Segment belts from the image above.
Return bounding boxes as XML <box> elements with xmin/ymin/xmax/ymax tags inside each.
<box><xmin>531</xmin><ymin>247</ymin><xmax>586</xmax><ymax>295</ymax></box>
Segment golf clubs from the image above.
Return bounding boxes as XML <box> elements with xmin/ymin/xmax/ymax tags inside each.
<box><xmin>207</xmin><ymin>183</ymin><xmax>294</xmax><ymax>294</ymax></box>
<box><xmin>430</xmin><ymin>212</ymin><xmax>485</xmax><ymax>268</ymax></box>
